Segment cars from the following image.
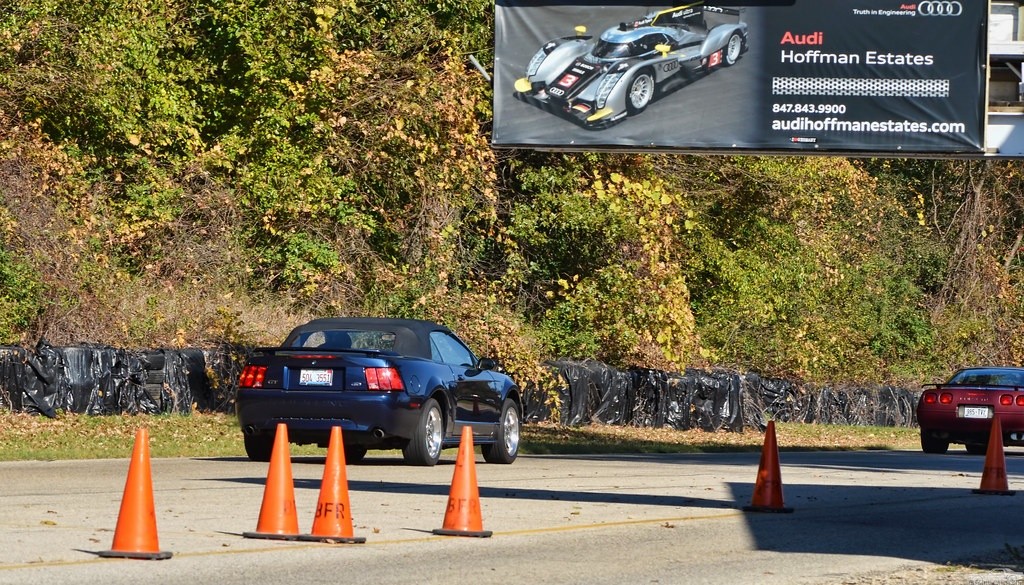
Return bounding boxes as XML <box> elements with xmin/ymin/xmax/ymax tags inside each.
<box><xmin>917</xmin><ymin>367</ymin><xmax>1024</xmax><ymax>455</ymax></box>
<box><xmin>514</xmin><ymin>1</ymin><xmax>750</xmax><ymax>130</ymax></box>
<box><xmin>235</xmin><ymin>317</ymin><xmax>523</xmax><ymax>466</ymax></box>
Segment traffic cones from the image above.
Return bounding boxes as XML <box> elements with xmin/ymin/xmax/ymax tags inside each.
<box><xmin>972</xmin><ymin>414</ymin><xmax>1016</xmax><ymax>496</ymax></box>
<box><xmin>97</xmin><ymin>428</ymin><xmax>173</xmax><ymax>559</ymax></box>
<box><xmin>303</xmin><ymin>426</ymin><xmax>367</xmax><ymax>544</ymax></box>
<box><xmin>243</xmin><ymin>424</ymin><xmax>304</xmax><ymax>541</ymax></box>
<box><xmin>742</xmin><ymin>421</ymin><xmax>794</xmax><ymax>514</ymax></box>
<box><xmin>433</xmin><ymin>426</ymin><xmax>492</xmax><ymax>538</ymax></box>
